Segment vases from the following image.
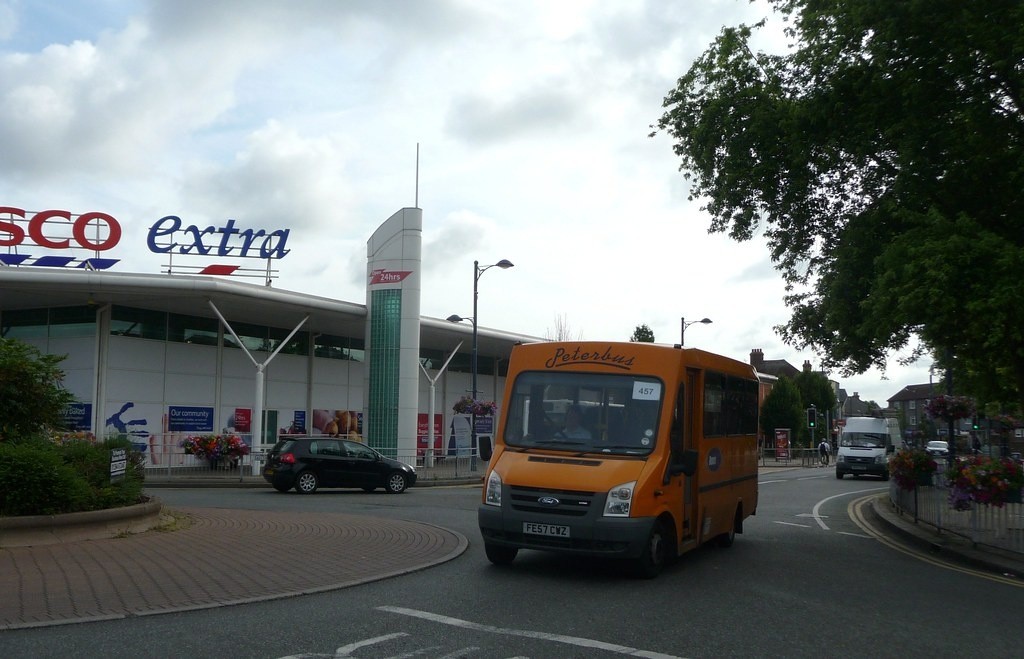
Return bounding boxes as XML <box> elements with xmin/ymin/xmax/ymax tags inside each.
<box><xmin>476</xmin><ymin>408</ymin><xmax>488</xmax><ymax>415</ymax></box>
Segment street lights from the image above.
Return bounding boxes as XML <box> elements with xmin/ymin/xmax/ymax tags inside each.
<box><xmin>682</xmin><ymin>319</ymin><xmax>713</xmax><ymax>345</ymax></box>
<box><xmin>445</xmin><ymin>259</ymin><xmax>515</xmax><ymax>470</ymax></box>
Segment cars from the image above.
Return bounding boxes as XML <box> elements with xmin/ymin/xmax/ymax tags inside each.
<box><xmin>902</xmin><ymin>440</ymin><xmax>910</xmax><ymax>450</ymax></box>
<box><xmin>263</xmin><ymin>433</ymin><xmax>418</xmax><ymax>495</ymax></box>
<box><xmin>926</xmin><ymin>441</ymin><xmax>951</xmax><ymax>458</ymax></box>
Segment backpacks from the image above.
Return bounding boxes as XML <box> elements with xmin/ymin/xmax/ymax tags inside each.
<box><xmin>820</xmin><ymin>443</ymin><xmax>825</xmax><ymax>453</ymax></box>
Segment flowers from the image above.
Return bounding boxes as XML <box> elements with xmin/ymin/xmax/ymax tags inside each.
<box><xmin>975</xmin><ymin>401</ymin><xmax>1022</xmax><ymax>436</ymax></box>
<box><xmin>886</xmin><ymin>448</ymin><xmax>937</xmax><ymax>493</ymax></box>
<box><xmin>941</xmin><ymin>452</ymin><xmax>1024</xmax><ymax>511</ymax></box>
<box><xmin>178</xmin><ymin>433</ymin><xmax>249</xmax><ymax>462</ymax></box>
<box><xmin>452</xmin><ymin>395</ymin><xmax>497</xmax><ymax>417</ymax></box>
<box><xmin>921</xmin><ymin>392</ymin><xmax>968</xmax><ymax>423</ymax></box>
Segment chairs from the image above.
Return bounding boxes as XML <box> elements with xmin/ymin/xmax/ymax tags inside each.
<box><xmin>542</xmin><ymin>402</ymin><xmax>621</xmax><ymax>442</ymax></box>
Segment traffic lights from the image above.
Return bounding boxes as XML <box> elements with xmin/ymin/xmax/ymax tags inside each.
<box><xmin>972</xmin><ymin>418</ymin><xmax>979</xmax><ymax>429</ymax></box>
<box><xmin>807</xmin><ymin>409</ymin><xmax>817</xmax><ymax>428</ymax></box>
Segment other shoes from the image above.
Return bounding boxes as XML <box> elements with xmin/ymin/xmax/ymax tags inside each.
<box><xmin>826</xmin><ymin>459</ymin><xmax>829</xmax><ymax>462</ymax></box>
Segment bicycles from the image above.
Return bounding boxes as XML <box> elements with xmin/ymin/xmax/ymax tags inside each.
<box><xmin>820</xmin><ymin>453</ymin><xmax>828</xmax><ymax>468</ymax></box>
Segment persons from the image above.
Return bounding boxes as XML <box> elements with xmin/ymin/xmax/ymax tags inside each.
<box><xmin>818</xmin><ymin>438</ymin><xmax>830</xmax><ymax>463</ymax></box>
<box><xmin>555</xmin><ymin>408</ymin><xmax>592</xmax><ymax>439</ymax></box>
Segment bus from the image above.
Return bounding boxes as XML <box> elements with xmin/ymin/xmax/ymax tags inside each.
<box><xmin>478</xmin><ymin>342</ymin><xmax>763</xmax><ymax>575</ymax></box>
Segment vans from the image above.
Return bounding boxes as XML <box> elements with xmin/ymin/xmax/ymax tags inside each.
<box><xmin>834</xmin><ymin>419</ymin><xmax>902</xmax><ymax>479</ymax></box>
